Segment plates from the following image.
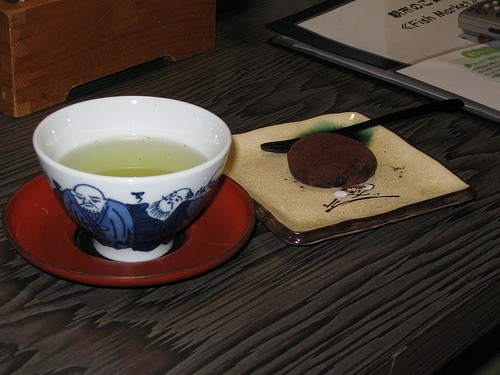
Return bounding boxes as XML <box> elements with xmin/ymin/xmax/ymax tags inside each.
<box><xmin>222</xmin><ymin>110</ymin><xmax>476</xmax><ymax>245</ymax></box>
<box><xmin>3</xmin><ymin>172</ymin><xmax>256</xmax><ymax>285</ymax></box>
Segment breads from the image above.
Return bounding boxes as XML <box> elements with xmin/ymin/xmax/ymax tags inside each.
<box><xmin>288</xmin><ymin>133</ymin><xmax>378</xmax><ymax>188</ymax></box>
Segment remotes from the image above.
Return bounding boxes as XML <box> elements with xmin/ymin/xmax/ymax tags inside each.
<box><xmin>457</xmin><ymin>0</ymin><xmax>500</xmax><ymax>41</ymax></box>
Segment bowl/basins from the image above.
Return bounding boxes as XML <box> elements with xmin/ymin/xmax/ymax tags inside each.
<box><xmin>32</xmin><ymin>95</ymin><xmax>232</xmax><ymax>263</ymax></box>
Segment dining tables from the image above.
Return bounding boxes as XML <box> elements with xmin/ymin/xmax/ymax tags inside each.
<box><xmin>0</xmin><ymin>0</ymin><xmax>500</xmax><ymax>375</ymax></box>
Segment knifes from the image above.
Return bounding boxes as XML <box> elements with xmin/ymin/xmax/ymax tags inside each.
<box><xmin>258</xmin><ymin>97</ymin><xmax>465</xmax><ymax>153</ymax></box>
